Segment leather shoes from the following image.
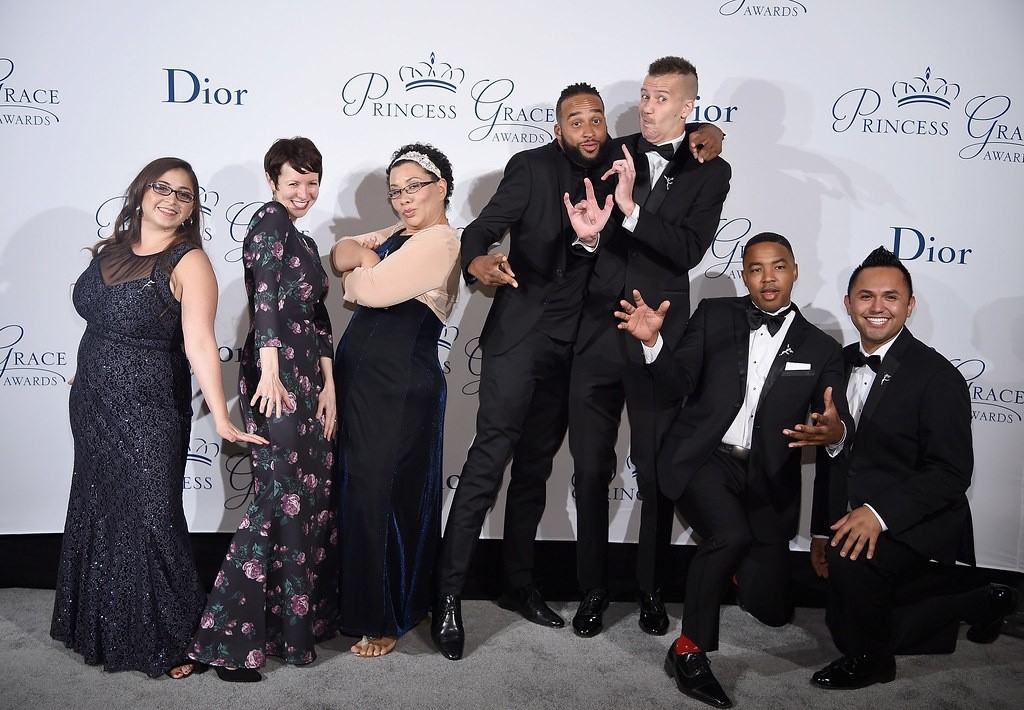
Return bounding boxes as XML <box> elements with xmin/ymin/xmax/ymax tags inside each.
<box><xmin>498</xmin><ymin>587</ymin><xmax>565</xmax><ymax>628</ymax></box>
<box><xmin>812</xmin><ymin>652</ymin><xmax>896</xmax><ymax>690</ymax></box>
<box><xmin>572</xmin><ymin>590</ymin><xmax>609</xmax><ymax>637</ymax></box>
<box><xmin>637</xmin><ymin>593</ymin><xmax>670</xmax><ymax>636</ymax></box>
<box><xmin>664</xmin><ymin>638</ymin><xmax>732</xmax><ymax>709</ymax></box>
<box><xmin>966</xmin><ymin>584</ymin><xmax>1018</xmax><ymax>644</ymax></box>
<box><xmin>432</xmin><ymin>593</ymin><xmax>464</xmax><ymax>660</ymax></box>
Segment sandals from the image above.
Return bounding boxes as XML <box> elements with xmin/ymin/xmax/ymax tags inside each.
<box><xmin>353</xmin><ymin>634</ymin><xmax>399</xmax><ymax>657</ymax></box>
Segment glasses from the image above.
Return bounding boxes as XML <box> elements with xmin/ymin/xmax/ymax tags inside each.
<box><xmin>148</xmin><ymin>182</ymin><xmax>196</xmax><ymax>203</ymax></box>
<box><xmin>387</xmin><ymin>179</ymin><xmax>439</xmax><ymax>199</ymax></box>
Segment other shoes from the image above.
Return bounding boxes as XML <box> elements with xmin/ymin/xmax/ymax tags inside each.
<box><xmin>163</xmin><ymin>661</ymin><xmax>195</xmax><ymax>679</ymax></box>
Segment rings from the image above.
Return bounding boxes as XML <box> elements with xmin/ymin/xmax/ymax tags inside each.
<box><xmin>334</xmin><ymin>420</ymin><xmax>336</xmax><ymax>423</ymax></box>
<box><xmin>262</xmin><ymin>396</ymin><xmax>268</xmax><ymax>399</ymax></box>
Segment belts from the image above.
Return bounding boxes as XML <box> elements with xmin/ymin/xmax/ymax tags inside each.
<box><xmin>717</xmin><ymin>442</ymin><xmax>751</xmax><ymax>464</ymax></box>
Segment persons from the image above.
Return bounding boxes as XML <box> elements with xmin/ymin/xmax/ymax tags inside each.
<box><xmin>187</xmin><ymin>137</ymin><xmax>340</xmax><ymax>683</ymax></box>
<box><xmin>331</xmin><ymin>144</ymin><xmax>462</xmax><ymax>657</ymax></box>
<box><xmin>428</xmin><ymin>82</ymin><xmax>723</xmax><ymax>661</ymax></box>
<box><xmin>808</xmin><ymin>247</ymin><xmax>978</xmax><ymax>689</ymax></box>
<box><xmin>562</xmin><ymin>55</ymin><xmax>732</xmax><ymax>638</ymax></box>
<box><xmin>49</xmin><ymin>156</ymin><xmax>269</xmax><ymax>678</ymax></box>
<box><xmin>613</xmin><ymin>232</ymin><xmax>855</xmax><ymax>709</ymax></box>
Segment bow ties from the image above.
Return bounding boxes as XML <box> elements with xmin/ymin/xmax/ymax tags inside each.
<box><xmin>637</xmin><ymin>137</ymin><xmax>674</xmax><ymax>161</ymax></box>
<box><xmin>851</xmin><ymin>351</ymin><xmax>881</xmax><ymax>374</ymax></box>
<box><xmin>745</xmin><ymin>304</ymin><xmax>792</xmax><ymax>337</ymax></box>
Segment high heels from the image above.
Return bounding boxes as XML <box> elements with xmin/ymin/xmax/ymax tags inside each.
<box><xmin>193</xmin><ymin>661</ymin><xmax>262</xmax><ymax>682</ymax></box>
